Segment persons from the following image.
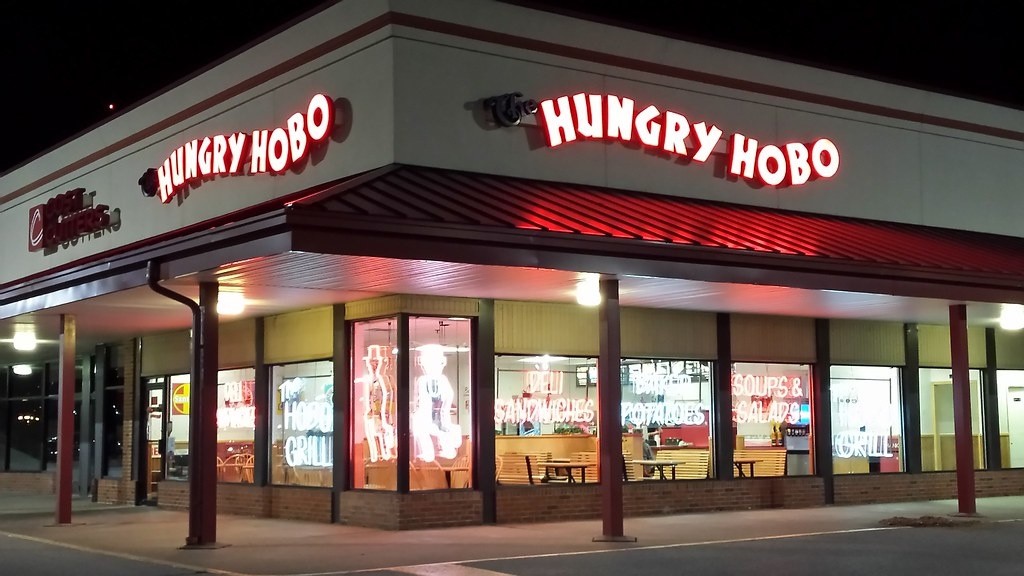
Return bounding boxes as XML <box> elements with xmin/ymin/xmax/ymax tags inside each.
<box><xmin>647</xmin><ymin>420</ymin><xmax>663</xmax><ymax>441</ymax></box>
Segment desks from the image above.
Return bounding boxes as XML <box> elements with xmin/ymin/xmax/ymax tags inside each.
<box><xmin>218</xmin><ymin>463</ymin><xmax>246</xmax><ymax>483</ymax></box>
<box><xmin>625</xmin><ymin>459</ymin><xmax>686</xmax><ymax>480</ymax></box>
<box><xmin>733</xmin><ymin>458</ymin><xmax>764</xmax><ymax>478</ymax></box>
<box><xmin>553</xmin><ymin>459</ymin><xmax>571</xmax><ymax>476</ymax></box>
<box><xmin>364</xmin><ymin>465</ymin><xmax>388</xmax><ymax>485</ymax></box>
<box><xmin>410</xmin><ymin>468</ymin><xmax>469</xmax><ymax>488</ymax></box>
<box><xmin>537</xmin><ymin>462</ymin><xmax>597</xmax><ymax>484</ymax></box>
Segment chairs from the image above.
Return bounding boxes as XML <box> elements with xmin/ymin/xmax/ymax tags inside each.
<box><xmin>495</xmin><ymin>456</ymin><xmax>504</xmax><ymax>482</ymax></box>
<box><xmin>409</xmin><ymin>456</ymin><xmax>471</xmax><ymax>488</ymax></box>
<box><xmin>217</xmin><ymin>453</ymin><xmax>254</xmax><ymax>482</ymax></box>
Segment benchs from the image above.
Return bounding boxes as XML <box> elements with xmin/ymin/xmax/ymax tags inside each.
<box><xmin>501</xmin><ymin>452</ymin><xmax>636</xmax><ymax>484</ymax></box>
<box><xmin>733</xmin><ymin>450</ymin><xmax>787</xmax><ymax>477</ymax></box>
<box><xmin>653</xmin><ymin>450</ymin><xmax>710</xmax><ymax>479</ymax></box>
